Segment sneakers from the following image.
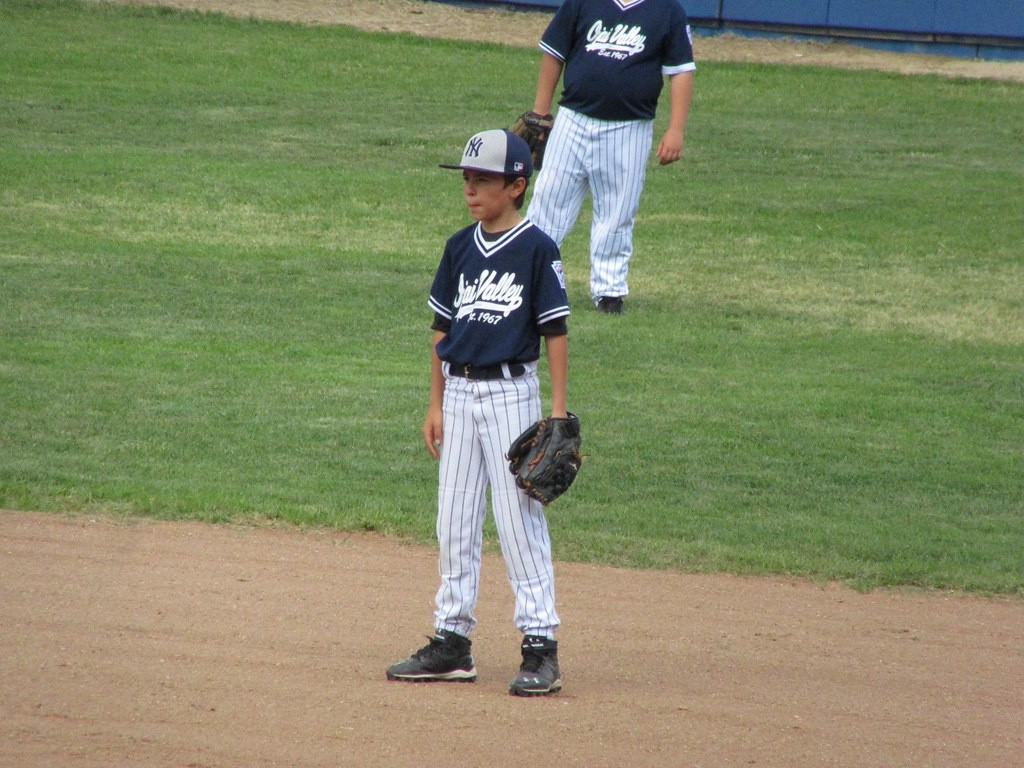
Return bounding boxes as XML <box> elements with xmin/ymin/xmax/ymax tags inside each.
<box><xmin>386</xmin><ymin>627</ymin><xmax>477</xmax><ymax>682</ymax></box>
<box><xmin>507</xmin><ymin>633</ymin><xmax>562</xmax><ymax>696</ymax></box>
<box><xmin>597</xmin><ymin>296</ymin><xmax>623</xmax><ymax>313</ymax></box>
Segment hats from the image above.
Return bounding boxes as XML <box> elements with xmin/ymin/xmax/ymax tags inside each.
<box><xmin>439</xmin><ymin>127</ymin><xmax>535</xmax><ymax>178</ymax></box>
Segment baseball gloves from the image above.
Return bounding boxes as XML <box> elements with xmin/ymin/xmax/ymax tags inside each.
<box><xmin>504</xmin><ymin>411</ymin><xmax>592</xmax><ymax>506</ymax></box>
<box><xmin>511</xmin><ymin>111</ymin><xmax>555</xmax><ymax>171</ymax></box>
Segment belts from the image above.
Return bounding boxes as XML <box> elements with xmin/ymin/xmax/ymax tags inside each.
<box><xmin>448</xmin><ymin>363</ymin><xmax>525</xmax><ymax>381</ymax></box>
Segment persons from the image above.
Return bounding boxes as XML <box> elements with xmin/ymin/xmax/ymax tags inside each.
<box><xmin>385</xmin><ymin>127</ymin><xmax>585</xmax><ymax>700</ymax></box>
<box><xmin>502</xmin><ymin>0</ymin><xmax>701</xmax><ymax>317</ymax></box>
